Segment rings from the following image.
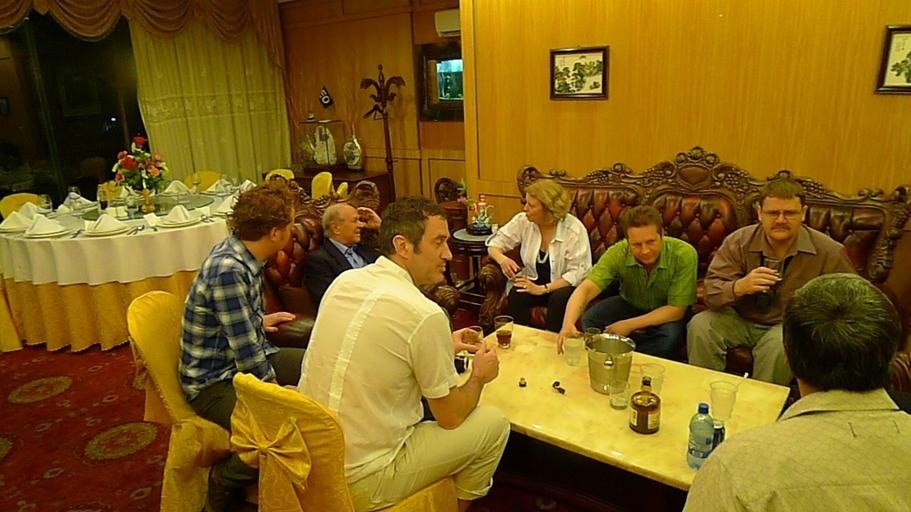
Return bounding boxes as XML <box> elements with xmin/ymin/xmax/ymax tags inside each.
<box><xmin>523</xmin><ymin>284</ymin><xmax>527</xmax><ymax>289</ymax></box>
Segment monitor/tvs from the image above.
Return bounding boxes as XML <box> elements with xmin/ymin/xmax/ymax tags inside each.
<box><xmin>427</xmin><ymin>56</ymin><xmax>463</xmax><ymax>102</ymax></box>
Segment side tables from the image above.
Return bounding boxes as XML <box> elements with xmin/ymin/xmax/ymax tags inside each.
<box><xmin>445</xmin><ymin>223</ymin><xmax>500</xmax><ymax>309</ymax></box>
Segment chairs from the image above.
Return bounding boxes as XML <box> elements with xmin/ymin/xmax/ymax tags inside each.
<box><xmin>98</xmin><ymin>181</ymin><xmax>127</xmax><ymax>201</ymax></box>
<box><xmin>311</xmin><ymin>172</ymin><xmax>336</xmax><ymax>199</ymax></box>
<box><xmin>0</xmin><ymin>193</ymin><xmax>53</xmax><ymax>219</ymax></box>
<box><xmin>183</xmin><ymin>172</ymin><xmax>220</xmax><ymax>190</ymax></box>
<box><xmin>337</xmin><ymin>182</ymin><xmax>348</xmax><ymax>199</ymax></box>
<box><xmin>264</xmin><ymin>169</ymin><xmax>294</xmax><ymax>182</ymax></box>
<box><xmin>434</xmin><ymin>177</ymin><xmax>468</xmax><ymax>229</ymax></box>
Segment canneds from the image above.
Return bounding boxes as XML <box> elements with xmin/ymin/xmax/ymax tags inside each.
<box><xmin>714</xmin><ymin>418</ymin><xmax>727</xmax><ymax>449</ymax></box>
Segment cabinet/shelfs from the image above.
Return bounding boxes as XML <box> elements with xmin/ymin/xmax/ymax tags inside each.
<box><xmin>261</xmin><ymin>171</ymin><xmax>393</xmax><ymax>214</ymax></box>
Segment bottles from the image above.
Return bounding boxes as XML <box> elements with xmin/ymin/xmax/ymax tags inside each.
<box><xmin>630</xmin><ymin>377</ymin><xmax>661</xmax><ymax>434</ymax></box>
<box><xmin>687</xmin><ymin>404</ymin><xmax>714</xmax><ymax>469</ymax></box>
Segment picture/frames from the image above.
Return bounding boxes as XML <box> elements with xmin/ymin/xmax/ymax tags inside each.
<box><xmin>875</xmin><ymin>23</ymin><xmax>911</xmax><ymax>95</ymax></box>
<box><xmin>550</xmin><ymin>44</ymin><xmax>608</xmax><ymax>100</ymax></box>
<box><xmin>414</xmin><ymin>42</ymin><xmax>464</xmax><ymax>122</ymax></box>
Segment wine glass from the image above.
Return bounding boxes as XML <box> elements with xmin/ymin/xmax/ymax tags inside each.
<box><xmin>36</xmin><ymin>173</ymin><xmax>228</xmax><ymax>212</ymax></box>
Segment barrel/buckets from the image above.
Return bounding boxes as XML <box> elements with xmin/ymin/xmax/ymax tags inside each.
<box><xmin>585</xmin><ymin>333</ymin><xmax>635</xmax><ymax>395</ymax></box>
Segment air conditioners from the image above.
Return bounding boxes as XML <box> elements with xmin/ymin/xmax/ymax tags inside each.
<box><xmin>433</xmin><ymin>8</ymin><xmax>459</xmax><ymax>38</ymax></box>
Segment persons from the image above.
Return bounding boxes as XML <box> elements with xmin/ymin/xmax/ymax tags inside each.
<box><xmin>300</xmin><ymin>202</ymin><xmax>386</xmax><ymax>308</ymax></box>
<box><xmin>557</xmin><ymin>205</ymin><xmax>698</xmax><ymax>364</ymax></box>
<box><xmin>178</xmin><ymin>179</ymin><xmax>307</xmax><ymax>512</ymax></box>
<box><xmin>486</xmin><ymin>178</ymin><xmax>593</xmax><ymax>331</ymax></box>
<box><xmin>687</xmin><ymin>177</ymin><xmax>860</xmax><ymax>383</ymax></box>
<box><xmin>684</xmin><ymin>274</ymin><xmax>911</xmax><ymax>511</ymax></box>
<box><xmin>297</xmin><ymin>194</ymin><xmax>511</xmax><ymax>512</ymax></box>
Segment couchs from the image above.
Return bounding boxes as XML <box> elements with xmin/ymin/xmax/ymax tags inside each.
<box><xmin>260</xmin><ymin>175</ymin><xmax>458</xmax><ymax>347</ymax></box>
<box><xmin>478</xmin><ymin>146</ymin><xmax>911</xmax><ymax>413</ymax></box>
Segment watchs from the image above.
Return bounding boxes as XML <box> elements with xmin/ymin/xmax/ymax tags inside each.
<box><xmin>544</xmin><ymin>282</ymin><xmax>550</xmax><ymax>293</ymax></box>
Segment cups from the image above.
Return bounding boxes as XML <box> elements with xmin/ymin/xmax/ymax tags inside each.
<box><xmin>494</xmin><ymin>316</ymin><xmax>513</xmax><ymax>348</ymax></box>
<box><xmin>640</xmin><ymin>364</ymin><xmax>665</xmax><ymax>393</ymax></box>
<box><xmin>585</xmin><ymin>327</ymin><xmax>602</xmax><ymax>347</ymax></box>
<box><xmin>563</xmin><ymin>332</ymin><xmax>584</xmax><ymax>366</ymax></box>
<box><xmin>765</xmin><ymin>255</ymin><xmax>785</xmax><ymax>276</ymax></box>
<box><xmin>462</xmin><ymin>326</ymin><xmax>484</xmax><ymax>354</ymax></box>
<box><xmin>610</xmin><ymin>380</ymin><xmax>629</xmax><ymax>408</ymax></box>
<box><xmin>709</xmin><ymin>381</ymin><xmax>737</xmax><ymax>422</ymax></box>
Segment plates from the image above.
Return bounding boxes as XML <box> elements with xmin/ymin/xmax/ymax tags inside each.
<box><xmin>0</xmin><ymin>189</ymin><xmax>235</xmax><ymax>239</ymax></box>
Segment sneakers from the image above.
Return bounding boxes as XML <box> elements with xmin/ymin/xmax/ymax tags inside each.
<box><xmin>205</xmin><ymin>465</ymin><xmax>230</xmax><ymax>512</ymax></box>
